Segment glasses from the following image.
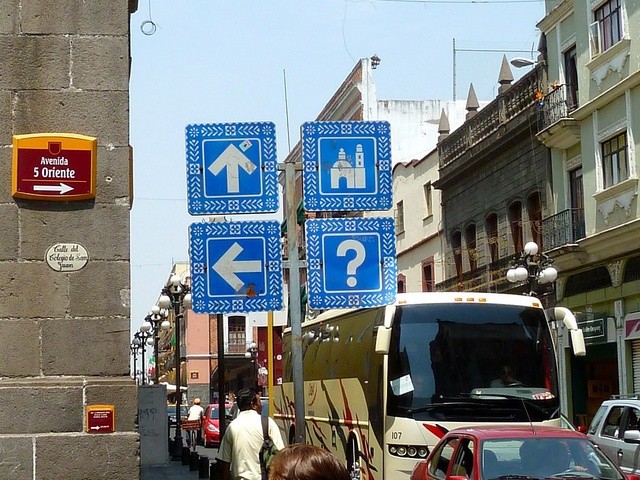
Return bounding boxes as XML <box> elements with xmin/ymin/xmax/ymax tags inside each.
<box><xmin>501</xmin><ymin>371</ymin><xmax>511</xmax><ymax>375</ymax></box>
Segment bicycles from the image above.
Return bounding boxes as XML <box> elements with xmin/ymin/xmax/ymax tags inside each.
<box><xmin>191</xmin><ymin>430</ymin><xmax>197</xmax><ymax>450</ymax></box>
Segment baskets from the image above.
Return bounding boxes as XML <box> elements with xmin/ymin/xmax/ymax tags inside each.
<box><xmin>180</xmin><ymin>420</ymin><xmax>200</xmax><ymax>429</ymax></box>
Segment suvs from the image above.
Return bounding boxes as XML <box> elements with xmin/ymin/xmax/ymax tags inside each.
<box><xmin>587</xmin><ymin>395</ymin><xmax>640</xmax><ymax>475</ymax></box>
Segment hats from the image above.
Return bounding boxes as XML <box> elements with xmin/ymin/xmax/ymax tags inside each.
<box><xmin>194</xmin><ymin>398</ymin><xmax>200</xmax><ymax>403</ymax></box>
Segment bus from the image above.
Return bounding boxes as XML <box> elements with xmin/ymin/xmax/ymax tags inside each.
<box><xmin>274</xmin><ymin>293</ymin><xmax>586</xmax><ymax>480</ymax></box>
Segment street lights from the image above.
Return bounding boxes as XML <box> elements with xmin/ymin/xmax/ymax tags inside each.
<box><xmin>131</xmin><ymin>333</ymin><xmax>139</xmax><ymax>379</ymax></box>
<box><xmin>507</xmin><ymin>242</ymin><xmax>557</xmax><ymax>295</ymax></box>
<box><xmin>159</xmin><ymin>275</ymin><xmax>192</xmax><ymax>456</ymax></box>
<box><xmin>140</xmin><ymin>326</ymin><xmax>150</xmax><ymax>384</ymax></box>
<box><xmin>146</xmin><ymin>306</ymin><xmax>172</xmax><ymax>383</ymax></box>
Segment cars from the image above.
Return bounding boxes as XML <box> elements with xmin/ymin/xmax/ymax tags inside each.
<box><xmin>202</xmin><ymin>404</ymin><xmax>232</xmax><ymax>447</ymax></box>
<box><xmin>168</xmin><ymin>406</ymin><xmax>188</xmax><ymax>425</ymax></box>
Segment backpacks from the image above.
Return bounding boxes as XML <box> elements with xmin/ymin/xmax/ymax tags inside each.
<box><xmin>259</xmin><ymin>415</ymin><xmax>278</xmax><ymax>480</ymax></box>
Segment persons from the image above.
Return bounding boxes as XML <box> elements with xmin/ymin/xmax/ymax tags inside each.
<box><xmin>538</xmin><ymin>441</ymin><xmax>586</xmax><ymax>475</ymax></box>
<box><xmin>215</xmin><ymin>388</ymin><xmax>285</xmax><ymax>480</ymax></box>
<box><xmin>186</xmin><ymin>398</ymin><xmax>204</xmax><ymax>446</ymax></box>
<box><xmin>268</xmin><ymin>443</ymin><xmax>352</xmax><ymax>480</ymax></box>
<box><xmin>490</xmin><ymin>364</ymin><xmax>523</xmax><ymax>388</ymax></box>
